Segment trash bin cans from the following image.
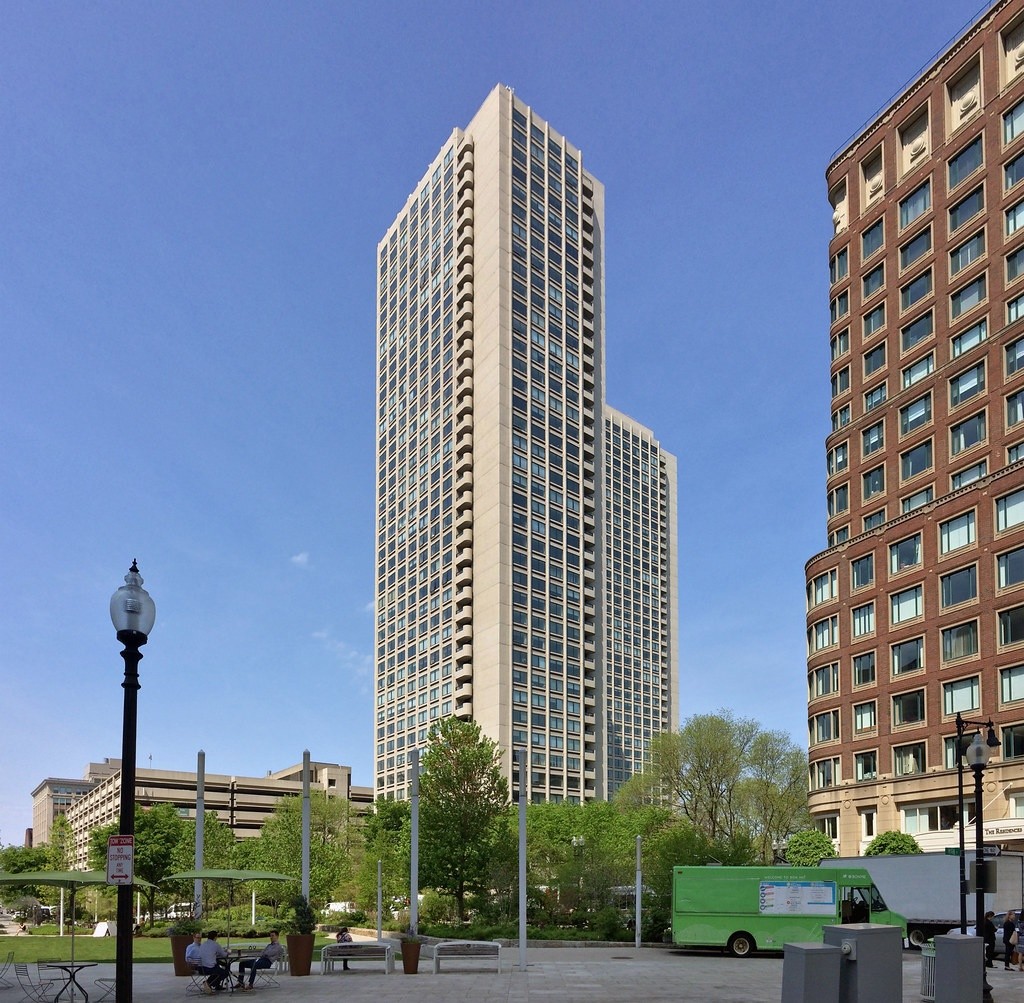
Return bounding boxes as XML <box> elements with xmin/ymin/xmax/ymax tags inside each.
<box><xmin>919</xmin><ymin>942</ymin><xmax>935</xmax><ymax>1002</ymax></box>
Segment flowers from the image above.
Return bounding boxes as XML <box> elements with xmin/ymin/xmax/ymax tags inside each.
<box><xmin>394</xmin><ymin>923</ymin><xmax>427</xmax><ymax>944</ymax></box>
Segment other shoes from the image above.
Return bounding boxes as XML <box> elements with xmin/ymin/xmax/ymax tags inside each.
<box><xmin>1005</xmin><ymin>967</ymin><xmax>1015</xmax><ymax>971</ymax></box>
<box><xmin>203</xmin><ymin>982</ymin><xmax>212</xmax><ymax>994</ymax></box>
<box><xmin>243</xmin><ymin>985</ymin><xmax>255</xmax><ymax>992</ymax></box>
<box><xmin>230</xmin><ymin>983</ymin><xmax>245</xmax><ymax>990</ymax></box>
<box><xmin>990</xmin><ymin>965</ymin><xmax>998</xmax><ymax>968</ymax></box>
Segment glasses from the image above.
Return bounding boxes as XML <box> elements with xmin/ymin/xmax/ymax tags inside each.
<box><xmin>194</xmin><ymin>937</ymin><xmax>201</xmax><ymax>939</ymax></box>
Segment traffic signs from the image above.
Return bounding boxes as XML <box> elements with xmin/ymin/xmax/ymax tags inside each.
<box><xmin>107</xmin><ymin>835</ymin><xmax>135</xmax><ymax>885</ymax></box>
<box><xmin>982</xmin><ymin>844</ymin><xmax>1002</xmax><ymax>857</ymax></box>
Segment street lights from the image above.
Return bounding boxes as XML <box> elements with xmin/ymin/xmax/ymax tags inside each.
<box><xmin>955</xmin><ymin>711</ymin><xmax>1002</xmax><ymax>939</ymax></box>
<box><xmin>965</xmin><ymin>727</ymin><xmax>995</xmax><ymax>1003</ymax></box>
<box><xmin>110</xmin><ymin>557</ymin><xmax>157</xmax><ymax>1002</ymax></box>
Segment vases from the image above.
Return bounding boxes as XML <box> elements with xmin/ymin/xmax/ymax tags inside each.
<box><xmin>400</xmin><ymin>944</ymin><xmax>420</xmax><ymax>974</ymax></box>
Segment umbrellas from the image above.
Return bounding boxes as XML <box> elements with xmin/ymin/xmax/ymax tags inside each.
<box><xmin>158</xmin><ymin>869</ymin><xmax>302</xmax><ymax>992</ymax></box>
<box><xmin>0</xmin><ymin>871</ymin><xmax>160</xmax><ymax>1003</ymax></box>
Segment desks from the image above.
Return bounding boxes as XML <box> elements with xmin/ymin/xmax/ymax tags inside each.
<box><xmin>47</xmin><ymin>961</ymin><xmax>98</xmax><ymax>1003</ymax></box>
<box><xmin>217</xmin><ymin>954</ymin><xmax>259</xmax><ymax>993</ymax></box>
<box><xmin>224</xmin><ymin>948</ymin><xmax>260</xmax><ymax>990</ymax></box>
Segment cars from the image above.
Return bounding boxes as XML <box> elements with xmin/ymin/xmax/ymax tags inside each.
<box><xmin>12</xmin><ymin>911</ymin><xmax>24</xmax><ymax>921</ymax></box>
<box><xmin>948</xmin><ymin>909</ymin><xmax>1024</xmax><ymax>959</ymax></box>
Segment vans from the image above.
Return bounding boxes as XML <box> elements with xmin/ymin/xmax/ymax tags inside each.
<box><xmin>321</xmin><ymin>902</ymin><xmax>357</xmax><ymax>916</ymax></box>
<box><xmin>164</xmin><ymin>902</ymin><xmax>195</xmax><ymax>919</ymax></box>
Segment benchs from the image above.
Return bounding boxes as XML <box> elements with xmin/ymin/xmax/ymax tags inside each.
<box><xmin>223</xmin><ymin>943</ymin><xmax>289</xmax><ymax>974</ymax></box>
<box><xmin>320</xmin><ymin>943</ymin><xmax>396</xmax><ymax>975</ymax></box>
<box><xmin>433</xmin><ymin>941</ymin><xmax>502</xmax><ymax>974</ymax></box>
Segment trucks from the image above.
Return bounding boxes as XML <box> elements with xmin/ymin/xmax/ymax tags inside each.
<box><xmin>672</xmin><ymin>865</ymin><xmax>908</xmax><ymax>957</ymax></box>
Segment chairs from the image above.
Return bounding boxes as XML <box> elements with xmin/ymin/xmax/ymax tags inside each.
<box><xmin>185</xmin><ymin>956</ymin><xmax>210</xmax><ymax>996</ymax></box>
<box><xmin>93</xmin><ymin>978</ymin><xmax>117</xmax><ymax>1003</ymax></box>
<box><xmin>14</xmin><ymin>964</ymin><xmax>54</xmax><ymax>1003</ymax></box>
<box><xmin>37</xmin><ymin>958</ymin><xmax>72</xmax><ymax>1003</ymax></box>
<box><xmin>0</xmin><ymin>952</ymin><xmax>14</xmax><ymax>987</ymax></box>
<box><xmin>244</xmin><ymin>948</ymin><xmax>284</xmax><ymax>989</ymax></box>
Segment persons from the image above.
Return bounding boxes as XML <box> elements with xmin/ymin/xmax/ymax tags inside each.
<box><xmin>16</xmin><ymin>922</ymin><xmax>25</xmax><ymax>936</ymax></box>
<box><xmin>197</xmin><ymin>930</ymin><xmax>229</xmax><ymax>994</ymax></box>
<box><xmin>336</xmin><ymin>928</ymin><xmax>352</xmax><ymax>970</ymax></box>
<box><xmin>185</xmin><ymin>931</ymin><xmax>226</xmax><ymax>991</ymax></box>
<box><xmin>985</xmin><ymin>911</ymin><xmax>999</xmax><ymax>968</ymax></box>
<box><xmin>1003</xmin><ymin>910</ymin><xmax>1024</xmax><ymax>971</ymax></box>
<box><xmin>230</xmin><ymin>930</ymin><xmax>283</xmax><ymax>992</ymax></box>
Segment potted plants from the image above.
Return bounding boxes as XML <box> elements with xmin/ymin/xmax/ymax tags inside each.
<box><xmin>286</xmin><ymin>884</ymin><xmax>317</xmax><ymax>976</ymax></box>
<box><xmin>166</xmin><ymin>894</ymin><xmax>205</xmax><ymax>976</ymax></box>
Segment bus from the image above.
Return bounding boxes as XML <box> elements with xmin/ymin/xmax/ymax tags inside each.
<box><xmin>32</xmin><ymin>905</ymin><xmax>59</xmax><ymax>918</ymax></box>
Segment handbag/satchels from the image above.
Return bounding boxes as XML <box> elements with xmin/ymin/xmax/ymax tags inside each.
<box><xmin>1011</xmin><ymin>944</ymin><xmax>1018</xmax><ymax>964</ymax></box>
<box><xmin>1009</xmin><ymin>930</ymin><xmax>1018</xmax><ymax>944</ymax></box>
